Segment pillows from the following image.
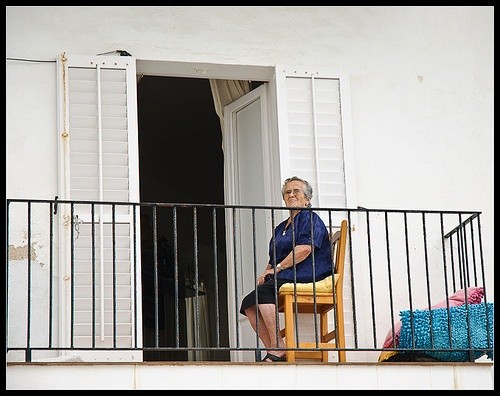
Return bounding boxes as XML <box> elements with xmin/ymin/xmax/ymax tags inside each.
<box><xmin>382</xmin><ymin>287</ymin><xmax>485</xmax><ymax>349</ymax></box>
<box><xmin>282</xmin><ymin>274</ymin><xmax>339</xmax><ymax>294</ymax></box>
<box><xmin>378</xmin><ymin>350</ymin><xmax>397</xmax><ymax>362</ymax></box>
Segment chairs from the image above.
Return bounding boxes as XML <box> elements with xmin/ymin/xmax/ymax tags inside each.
<box><xmin>277</xmin><ymin>221</ymin><xmax>348</xmax><ymax>364</ymax></box>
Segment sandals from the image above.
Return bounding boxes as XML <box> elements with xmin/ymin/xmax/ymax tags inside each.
<box><xmin>261</xmin><ymin>352</ymin><xmax>286</xmax><ymax>362</ymax></box>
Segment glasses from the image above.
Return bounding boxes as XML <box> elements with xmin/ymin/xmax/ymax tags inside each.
<box><xmin>285</xmin><ymin>190</ymin><xmax>311</xmax><ymax>200</ymax></box>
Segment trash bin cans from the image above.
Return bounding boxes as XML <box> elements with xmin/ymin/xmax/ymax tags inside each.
<box><xmin>168</xmin><ymin>276</ymin><xmax>211</xmax><ymax>361</ymax></box>
